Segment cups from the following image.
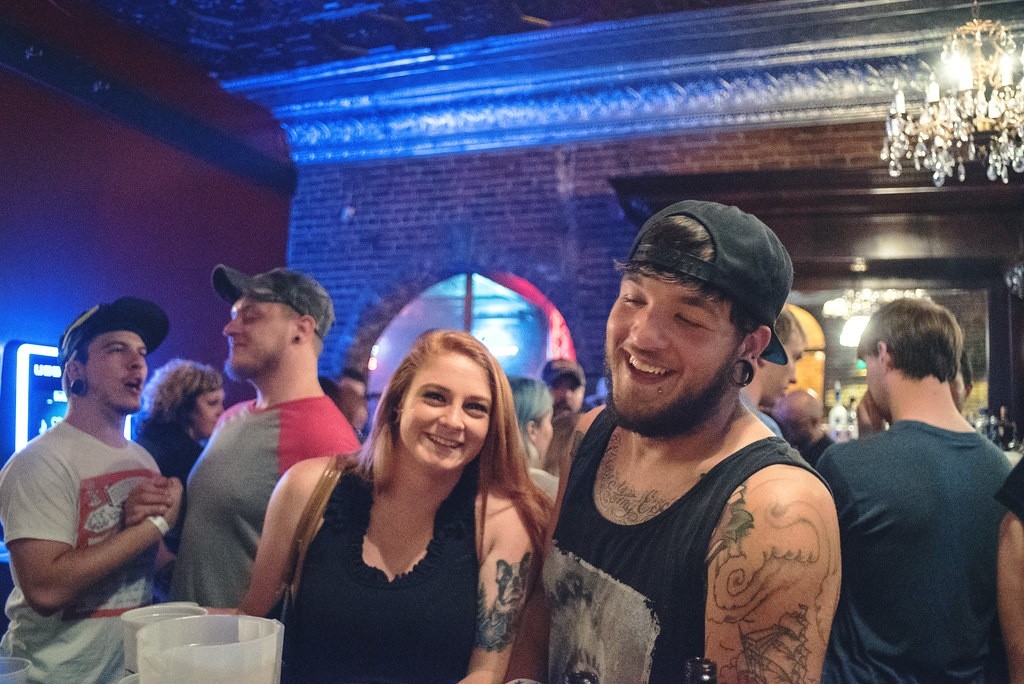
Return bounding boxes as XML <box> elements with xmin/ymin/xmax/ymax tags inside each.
<box><xmin>121</xmin><ymin>604</ymin><xmax>209</xmax><ymax>676</ymax></box>
<box><xmin>0</xmin><ymin>656</ymin><xmax>33</xmax><ymax>684</ymax></box>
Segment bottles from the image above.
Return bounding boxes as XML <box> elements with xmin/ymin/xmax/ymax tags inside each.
<box><xmin>685</xmin><ymin>657</ymin><xmax>717</xmax><ymax>684</ymax></box>
<box><xmin>568</xmin><ymin>672</ymin><xmax>598</xmax><ymax>684</ymax></box>
<box><xmin>829</xmin><ymin>380</ymin><xmax>858</xmax><ymax>442</ymax></box>
<box><xmin>975</xmin><ymin>406</ymin><xmax>1015</xmax><ymax>451</ymax></box>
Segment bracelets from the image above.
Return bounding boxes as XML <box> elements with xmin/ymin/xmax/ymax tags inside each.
<box><xmin>145</xmin><ymin>513</ymin><xmax>169</xmax><ymax>535</ymax></box>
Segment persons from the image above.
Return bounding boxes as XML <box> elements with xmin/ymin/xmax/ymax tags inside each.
<box><xmin>123</xmin><ymin>264</ymin><xmax>359</xmax><ymax>606</ymax></box>
<box><xmin>502</xmin><ymin>200</ymin><xmax>841</xmax><ymax>684</ymax></box>
<box><xmin>0</xmin><ymin>298</ymin><xmax>182</xmax><ymax>684</ymax></box>
<box><xmin>741</xmin><ymin>308</ymin><xmax>973</xmax><ymax>467</ymax></box>
<box><xmin>996</xmin><ymin>456</ymin><xmax>1024</xmax><ymax>684</ymax></box>
<box><xmin>210</xmin><ymin>327</ymin><xmax>550</xmax><ymax>684</ymax></box>
<box><xmin>507</xmin><ymin>358</ymin><xmax>587</xmax><ymax>499</ymax></box>
<box><xmin>815</xmin><ymin>298</ymin><xmax>1012</xmax><ymax>684</ymax></box>
<box><xmin>318</xmin><ymin>367</ymin><xmax>372</xmax><ymax>442</ymax></box>
<box><xmin>133</xmin><ymin>363</ymin><xmax>226</xmax><ymax>484</ymax></box>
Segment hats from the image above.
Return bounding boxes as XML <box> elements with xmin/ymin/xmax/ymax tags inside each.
<box><xmin>542</xmin><ymin>358</ymin><xmax>587</xmax><ymax>387</ymax></box>
<box><xmin>211</xmin><ymin>264</ymin><xmax>335</xmax><ymax>339</ymax></box>
<box><xmin>57</xmin><ymin>295</ymin><xmax>170</xmax><ymax>375</ymax></box>
<box><xmin>628</xmin><ymin>201</ymin><xmax>795</xmax><ymax>365</ymax></box>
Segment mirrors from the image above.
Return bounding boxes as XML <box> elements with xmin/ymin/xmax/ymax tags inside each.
<box><xmin>787</xmin><ymin>259</ymin><xmax>1013</xmax><ymax>419</ymax></box>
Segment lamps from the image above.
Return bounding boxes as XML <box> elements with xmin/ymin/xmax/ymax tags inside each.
<box><xmin>880</xmin><ymin>0</ymin><xmax>1024</xmax><ymax>187</ymax></box>
<box><xmin>822</xmin><ymin>289</ymin><xmax>932</xmax><ymax>347</ymax></box>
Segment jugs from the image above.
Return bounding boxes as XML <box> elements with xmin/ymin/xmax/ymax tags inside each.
<box><xmin>138</xmin><ymin>616</ymin><xmax>286</xmax><ymax>684</ymax></box>
